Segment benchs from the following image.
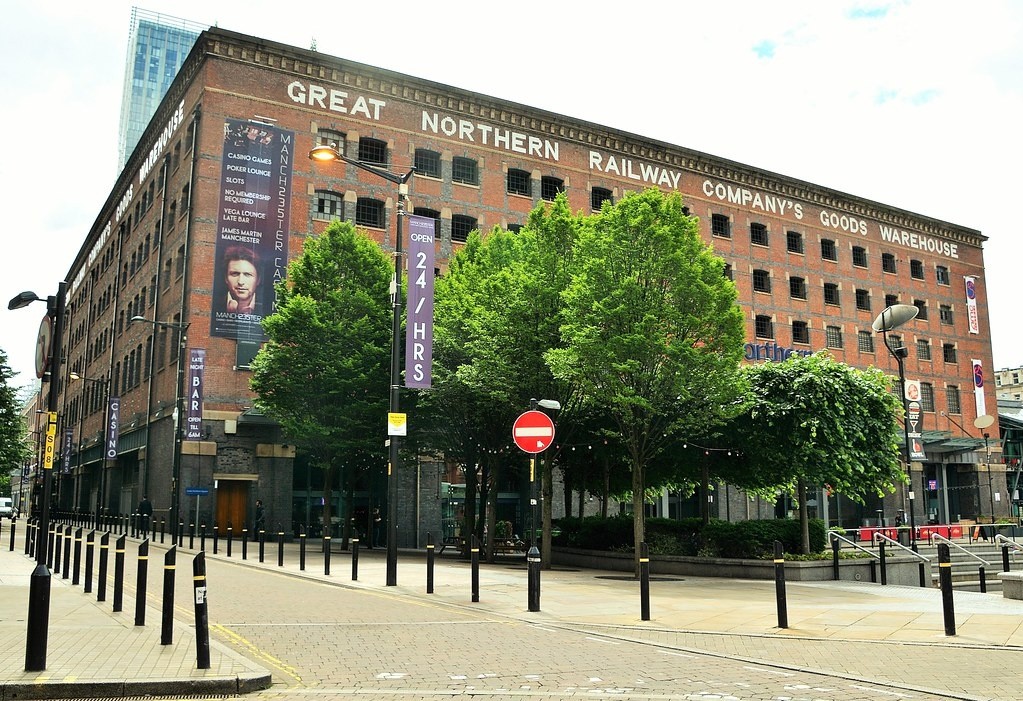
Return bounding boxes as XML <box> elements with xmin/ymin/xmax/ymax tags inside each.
<box><xmin>439</xmin><ymin>544</ymin><xmax>532</xmax><ymax>563</ymax></box>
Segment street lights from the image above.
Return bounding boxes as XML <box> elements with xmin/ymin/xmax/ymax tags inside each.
<box><xmin>524</xmin><ymin>396</ymin><xmax>560</xmax><ymax>613</ymax></box>
<box><xmin>130</xmin><ymin>314</ymin><xmax>187</xmax><ymax>546</ymax></box>
<box><xmin>973</xmin><ymin>415</ymin><xmax>996</xmax><ymax>544</ymax></box>
<box><xmin>307</xmin><ymin>145</ymin><xmax>417</xmax><ymax>587</ymax></box>
<box><xmin>35</xmin><ymin>409</ymin><xmax>67</xmax><ymax>510</ymax></box>
<box><xmin>69</xmin><ymin>372</ymin><xmax>109</xmax><ymax>531</ymax></box>
<box><xmin>872</xmin><ymin>303</ymin><xmax>920</xmax><ymax>559</ymax></box>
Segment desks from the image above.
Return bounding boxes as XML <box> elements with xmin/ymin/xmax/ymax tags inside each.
<box><xmin>493</xmin><ymin>537</ymin><xmax>521</xmax><ymax>557</ymax></box>
<box><xmin>448</xmin><ymin>536</ymin><xmax>482</xmax><ymax>556</ymax></box>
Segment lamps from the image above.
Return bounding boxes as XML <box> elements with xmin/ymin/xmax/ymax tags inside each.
<box><xmin>69</xmin><ymin>371</ymin><xmax>109</xmax><ymax>386</ymax></box>
<box><xmin>32</xmin><ymin>409</ymin><xmax>65</xmax><ymax>418</ymax></box>
<box><xmin>131</xmin><ymin>315</ymin><xmax>191</xmax><ymax>332</ymax></box>
<box><xmin>7</xmin><ymin>291</ymin><xmax>50</xmax><ymax>310</ymax></box>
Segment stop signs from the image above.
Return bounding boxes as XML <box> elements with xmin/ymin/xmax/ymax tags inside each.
<box><xmin>512</xmin><ymin>411</ymin><xmax>553</xmax><ymax>452</ymax></box>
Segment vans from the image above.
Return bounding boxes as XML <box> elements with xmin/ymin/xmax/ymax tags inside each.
<box><xmin>0</xmin><ymin>497</ymin><xmax>13</xmax><ymax>520</ymax></box>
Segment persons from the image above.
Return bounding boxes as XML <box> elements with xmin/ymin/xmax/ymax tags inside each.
<box><xmin>895</xmin><ymin>512</ymin><xmax>902</xmax><ymax>527</ymax></box>
<box><xmin>224</xmin><ymin>246</ymin><xmax>263</xmax><ymax>316</ymax></box>
<box><xmin>138</xmin><ymin>494</ymin><xmax>152</xmax><ymax>535</ymax></box>
<box><xmin>252</xmin><ymin>500</ymin><xmax>265</xmax><ymax>541</ymax></box>
